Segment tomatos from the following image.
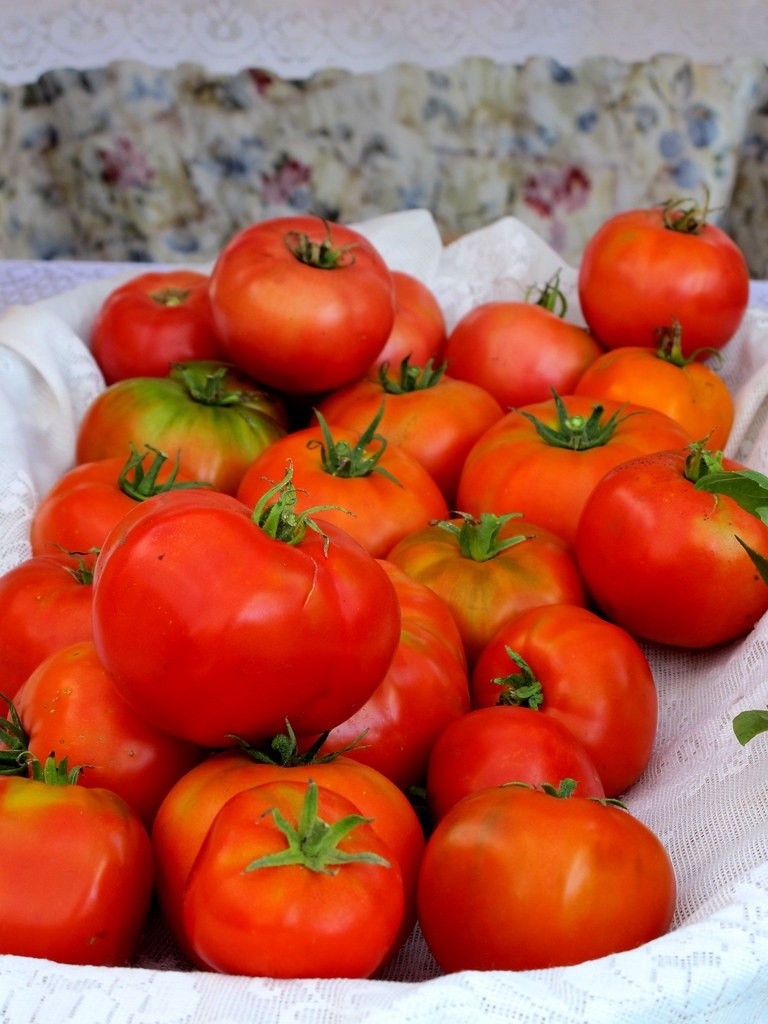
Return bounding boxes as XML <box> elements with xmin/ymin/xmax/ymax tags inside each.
<box><xmin>0</xmin><ymin>208</ymin><xmax>768</xmax><ymax>979</ymax></box>
